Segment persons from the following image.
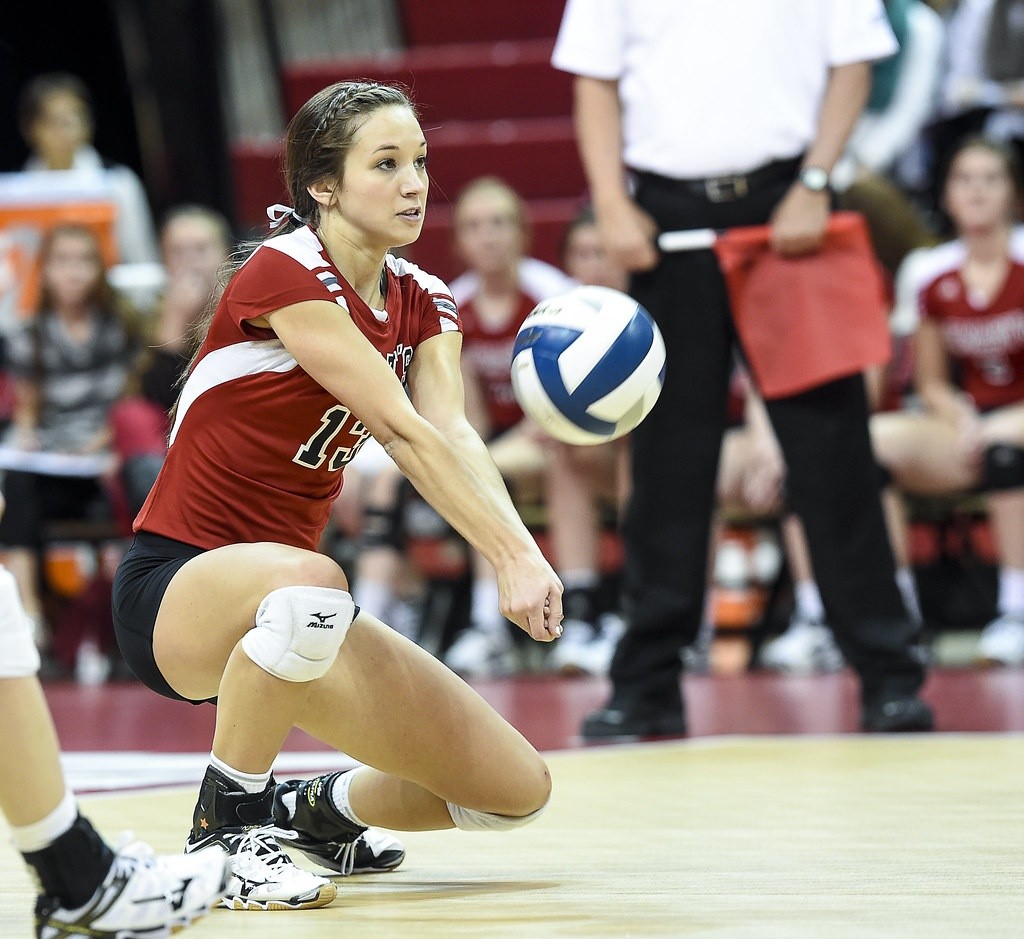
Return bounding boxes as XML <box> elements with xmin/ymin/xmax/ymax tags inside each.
<box><xmin>110</xmin><ymin>78</ymin><xmax>564</xmax><ymax>912</ymax></box>
<box><xmin>550</xmin><ymin>0</ymin><xmax>938</xmax><ymax>739</ymax></box>
<box><xmin>0</xmin><ymin>522</ymin><xmax>230</xmax><ymax>939</ymax></box>
<box><xmin>0</xmin><ymin>0</ymin><xmax>1024</xmax><ymax>701</ymax></box>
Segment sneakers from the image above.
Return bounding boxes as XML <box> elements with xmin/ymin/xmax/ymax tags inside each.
<box><xmin>271</xmin><ymin>779</ymin><xmax>405</xmax><ymax>876</ymax></box>
<box><xmin>184</xmin><ymin>782</ymin><xmax>338</xmax><ymax>911</ymax></box>
<box><xmin>34</xmin><ymin>838</ymin><xmax>231</xmax><ymax>939</ymax></box>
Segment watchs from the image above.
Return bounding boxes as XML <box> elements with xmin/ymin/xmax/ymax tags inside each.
<box><xmin>798</xmin><ymin>164</ymin><xmax>831</xmax><ymax>193</ymax></box>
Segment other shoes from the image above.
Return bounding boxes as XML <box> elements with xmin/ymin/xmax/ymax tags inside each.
<box><xmin>761</xmin><ymin>620</ymin><xmax>843</xmax><ymax>673</ymax></box>
<box><xmin>444</xmin><ymin>629</ymin><xmax>514</xmax><ymax>671</ymax></box>
<box><xmin>979</xmin><ymin>616</ymin><xmax>1024</xmax><ymax>663</ymax></box>
<box><xmin>552</xmin><ymin>621</ymin><xmax>594</xmax><ymax>668</ymax></box>
<box><xmin>562</xmin><ymin>635</ymin><xmax>616</xmax><ymax>673</ymax></box>
<box><xmin>580</xmin><ymin>700</ymin><xmax>688</xmax><ymax>740</ymax></box>
<box><xmin>858</xmin><ymin>695</ymin><xmax>932</xmax><ymax>728</ymax></box>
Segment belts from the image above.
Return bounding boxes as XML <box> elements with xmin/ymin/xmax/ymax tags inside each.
<box><xmin>635</xmin><ymin>158</ymin><xmax>802</xmax><ymax>201</ymax></box>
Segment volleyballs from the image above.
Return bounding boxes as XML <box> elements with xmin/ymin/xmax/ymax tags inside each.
<box><xmin>510</xmin><ymin>285</ymin><xmax>666</xmax><ymax>446</ymax></box>
